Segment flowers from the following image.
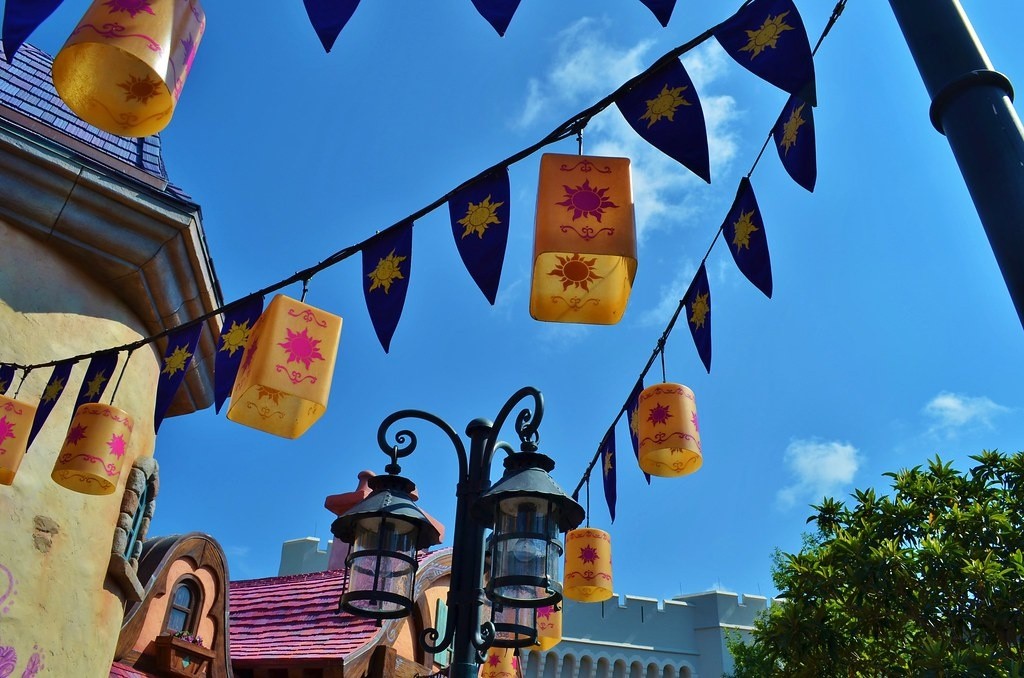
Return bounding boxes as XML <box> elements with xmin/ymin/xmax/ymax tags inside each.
<box><xmin>170</xmin><ymin>630</ymin><xmax>204</xmax><ymax>648</ymax></box>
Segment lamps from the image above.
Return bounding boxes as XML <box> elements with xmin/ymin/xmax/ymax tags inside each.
<box><xmin>50</xmin><ymin>403</ymin><xmax>135</xmax><ymax>495</ymax></box>
<box><xmin>50</xmin><ymin>0</ymin><xmax>205</xmax><ymax>138</ymax></box>
<box><xmin>563</xmin><ymin>529</ymin><xmax>613</xmax><ymax>603</ymax></box>
<box><xmin>0</xmin><ymin>393</ymin><xmax>37</xmax><ymax>485</ymax></box>
<box><xmin>226</xmin><ymin>294</ymin><xmax>343</xmax><ymax>439</ymax></box>
<box><xmin>637</xmin><ymin>383</ymin><xmax>702</xmax><ymax>478</ymax></box>
<box><xmin>481</xmin><ymin>638</ymin><xmax>517</xmax><ymax>678</ymax></box>
<box><xmin>529</xmin><ymin>152</ymin><xmax>638</xmax><ymax>324</ymax></box>
<box><xmin>510</xmin><ymin>583</ymin><xmax>563</xmax><ymax>653</ymax></box>
